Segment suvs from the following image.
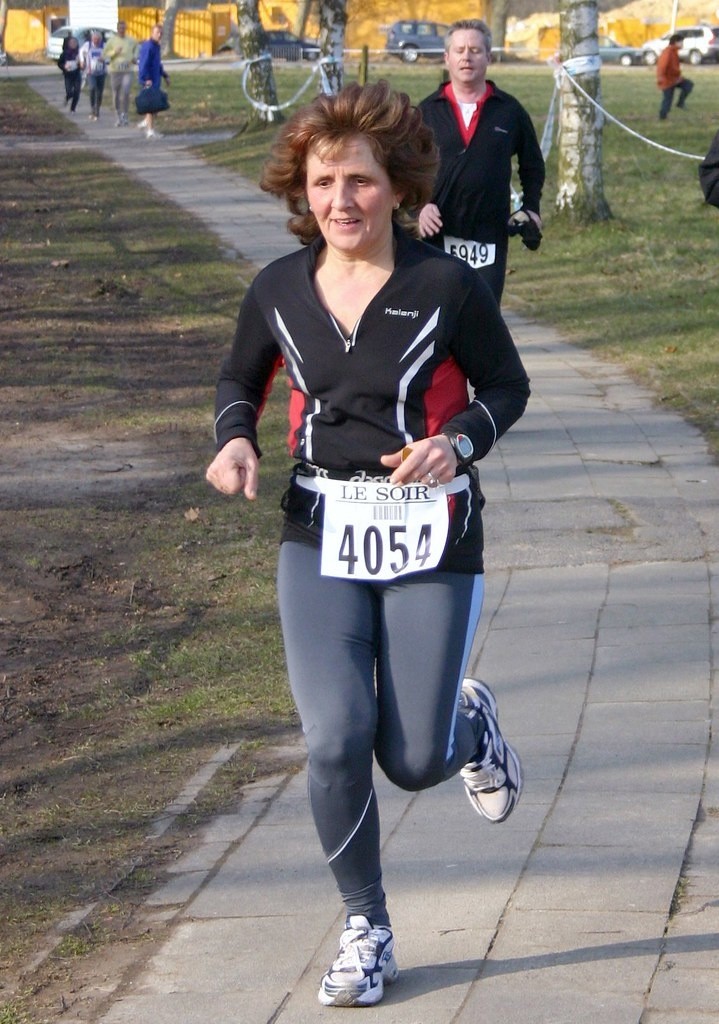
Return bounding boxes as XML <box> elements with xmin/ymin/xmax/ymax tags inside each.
<box><xmin>640</xmin><ymin>26</ymin><xmax>719</xmax><ymax>65</ymax></box>
<box><xmin>382</xmin><ymin>20</ymin><xmax>455</xmax><ymax>64</ymax></box>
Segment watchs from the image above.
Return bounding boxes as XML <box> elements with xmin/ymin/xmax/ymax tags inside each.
<box><xmin>443</xmin><ymin>430</ymin><xmax>474</xmax><ymax>467</ymax></box>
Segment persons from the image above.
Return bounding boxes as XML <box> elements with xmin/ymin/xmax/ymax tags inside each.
<box><xmin>58</xmin><ymin>36</ymin><xmax>84</xmax><ymax>116</ymax></box>
<box><xmin>137</xmin><ymin>24</ymin><xmax>170</xmax><ymax>139</ymax></box>
<box><xmin>657</xmin><ymin>34</ymin><xmax>693</xmax><ymax>119</ymax></box>
<box><xmin>405</xmin><ymin>19</ymin><xmax>545</xmax><ymax>307</ymax></box>
<box><xmin>78</xmin><ymin>28</ymin><xmax>110</xmax><ymax>121</ymax></box>
<box><xmin>204</xmin><ymin>82</ymin><xmax>530</xmax><ymax>1007</ymax></box>
<box><xmin>99</xmin><ymin>21</ymin><xmax>138</xmax><ymax>127</ymax></box>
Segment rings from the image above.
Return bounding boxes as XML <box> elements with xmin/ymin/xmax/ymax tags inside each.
<box><xmin>427</xmin><ymin>473</ymin><xmax>436</xmax><ymax>484</ymax></box>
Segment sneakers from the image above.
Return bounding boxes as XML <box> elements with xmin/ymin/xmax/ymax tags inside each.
<box><xmin>319</xmin><ymin>915</ymin><xmax>400</xmax><ymax>1008</ymax></box>
<box><xmin>456</xmin><ymin>679</ymin><xmax>524</xmax><ymax>826</ymax></box>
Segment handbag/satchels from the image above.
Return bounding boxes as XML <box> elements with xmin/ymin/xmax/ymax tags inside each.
<box><xmin>134</xmin><ymin>84</ymin><xmax>169</xmax><ymax>115</ymax></box>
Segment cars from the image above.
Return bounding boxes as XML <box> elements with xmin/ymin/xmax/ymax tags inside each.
<box><xmin>44</xmin><ymin>25</ymin><xmax>120</xmax><ymax>63</ymax></box>
<box><xmin>594</xmin><ymin>33</ymin><xmax>644</xmax><ymax>68</ymax></box>
<box><xmin>228</xmin><ymin>29</ymin><xmax>322</xmax><ymax>64</ymax></box>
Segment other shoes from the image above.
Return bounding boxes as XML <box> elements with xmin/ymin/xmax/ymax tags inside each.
<box><xmin>138</xmin><ymin>121</ymin><xmax>147</xmax><ymax>129</ymax></box>
<box><xmin>146</xmin><ymin>130</ymin><xmax>165</xmax><ymax>140</ymax></box>
<box><xmin>116</xmin><ymin>115</ymin><xmax>129</xmax><ymax>128</ymax></box>
